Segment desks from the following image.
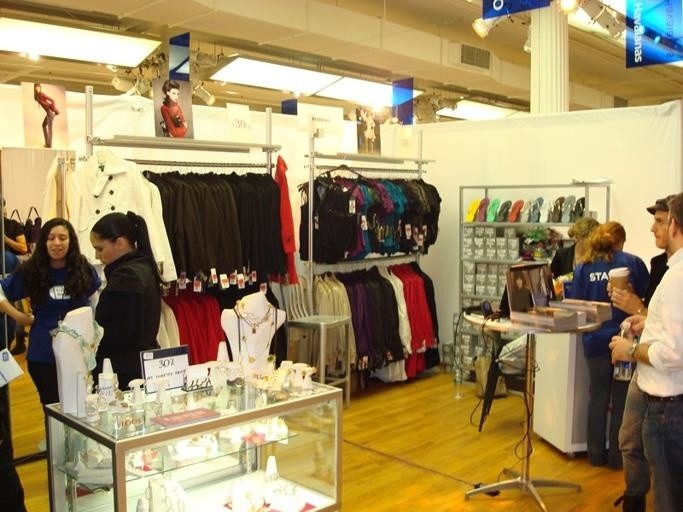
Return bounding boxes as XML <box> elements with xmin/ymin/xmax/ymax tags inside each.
<box><xmin>461</xmin><ymin>306</ymin><xmax>603</xmax><ymax>512</ymax></box>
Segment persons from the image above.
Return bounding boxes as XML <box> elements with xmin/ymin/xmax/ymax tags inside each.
<box><xmin>90</xmin><ymin>212</ymin><xmax>162</xmax><ymax>392</ymax></box>
<box><xmin>513</xmin><ymin>271</ymin><xmax>531</xmax><ymax>311</ymax></box>
<box><xmin>2</xmin><ymin>200</ymin><xmax>28</xmax><ymax>354</ymax></box>
<box><xmin>0</xmin><ymin>219</ymin><xmax>103</xmax><ymax>407</ymax></box>
<box><xmin>537</xmin><ymin>267</ymin><xmax>549</xmax><ymax>300</ymax></box>
<box><xmin>160</xmin><ymin>80</ymin><xmax>188</xmax><ymax>137</ymax></box>
<box><xmin>35</xmin><ymin>84</ymin><xmax>58</xmax><ymax>149</ymax></box>
<box><xmin>552</xmin><ymin>218</ymin><xmax>599</xmax><ymax>284</ymax></box>
<box><xmin>572</xmin><ymin>221</ymin><xmax>649</xmax><ymax>465</ymax></box>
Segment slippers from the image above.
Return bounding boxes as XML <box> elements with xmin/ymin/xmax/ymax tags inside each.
<box><xmin>477</xmin><ymin>198</ymin><xmax>489</xmax><ymax>222</ymax></box>
<box><xmin>561</xmin><ymin>195</ymin><xmax>576</xmax><ymax>224</ymax></box>
<box><xmin>530</xmin><ymin>197</ymin><xmax>544</xmax><ymax>223</ymax></box>
<box><xmin>465</xmin><ymin>197</ymin><xmax>482</xmax><ymax>222</ymax></box>
<box><xmin>573</xmin><ymin>197</ymin><xmax>585</xmax><ymax>224</ymax></box>
<box><xmin>552</xmin><ymin>197</ymin><xmax>565</xmax><ymax>223</ymax></box>
<box><xmin>485</xmin><ymin>198</ymin><xmax>500</xmax><ymax>222</ymax></box>
<box><xmin>508</xmin><ymin>200</ymin><xmax>524</xmax><ymax>222</ymax></box>
<box><xmin>498</xmin><ymin>200</ymin><xmax>512</xmax><ymax>222</ymax></box>
<box><xmin>521</xmin><ymin>199</ymin><xmax>533</xmax><ymax>223</ymax></box>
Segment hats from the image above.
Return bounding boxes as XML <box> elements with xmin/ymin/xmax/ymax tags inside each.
<box><xmin>646</xmin><ymin>196</ymin><xmax>675</xmax><ymax>214</ymax></box>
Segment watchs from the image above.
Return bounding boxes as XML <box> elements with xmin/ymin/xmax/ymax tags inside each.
<box><xmin>627</xmin><ymin>343</ymin><xmax>638</xmax><ymax>358</ymax></box>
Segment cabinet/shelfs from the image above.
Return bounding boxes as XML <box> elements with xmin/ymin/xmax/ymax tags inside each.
<box><xmin>84</xmin><ymin>132</ymin><xmax>283</xmax><ymax>292</ymax></box>
<box><xmin>43</xmin><ymin>380</ymin><xmax>344</xmax><ymax>512</ymax></box>
<box><xmin>457</xmin><ymin>180</ymin><xmax>612</xmax><ymax>400</ymax></box>
<box><xmin>303</xmin><ymin>149</ymin><xmax>437</xmax><ymax>365</ymax></box>
<box><xmin>530</xmin><ymin>332</ymin><xmax>624</xmax><ymax>460</ymax></box>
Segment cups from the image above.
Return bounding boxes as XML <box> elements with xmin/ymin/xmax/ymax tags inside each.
<box><xmin>609</xmin><ymin>268</ymin><xmax>629</xmax><ymax>291</ymax></box>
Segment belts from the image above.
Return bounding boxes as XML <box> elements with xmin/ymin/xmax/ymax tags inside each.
<box><xmin>641</xmin><ymin>394</ymin><xmax>682</xmax><ymax>403</ymax></box>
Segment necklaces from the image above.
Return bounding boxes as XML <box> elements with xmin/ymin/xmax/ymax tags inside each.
<box><xmin>235</xmin><ymin>305</ymin><xmax>275</xmax><ymax>365</ymax></box>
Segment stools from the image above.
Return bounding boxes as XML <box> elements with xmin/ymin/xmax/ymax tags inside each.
<box><xmin>284</xmin><ymin>314</ymin><xmax>354</xmax><ymax>410</ymax></box>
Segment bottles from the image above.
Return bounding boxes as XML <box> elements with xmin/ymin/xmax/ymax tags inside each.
<box><xmin>612</xmin><ymin>322</ymin><xmax>632</xmax><ymax>382</ymax></box>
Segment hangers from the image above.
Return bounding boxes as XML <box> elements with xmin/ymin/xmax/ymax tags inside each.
<box><xmin>317</xmin><ymin>153</ymin><xmax>364</xmax><ymax>180</ymax></box>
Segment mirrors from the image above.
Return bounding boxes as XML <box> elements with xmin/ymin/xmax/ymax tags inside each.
<box><xmin>0</xmin><ymin>146</ymin><xmax>81</xmax><ymax>468</ymax></box>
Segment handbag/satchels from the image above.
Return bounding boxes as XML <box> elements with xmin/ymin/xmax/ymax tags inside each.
<box><xmin>8</xmin><ymin>209</ymin><xmax>24</xmax><ymax>228</ymax></box>
<box><xmin>24</xmin><ymin>206</ymin><xmax>43</xmax><ymax>244</ymax></box>
